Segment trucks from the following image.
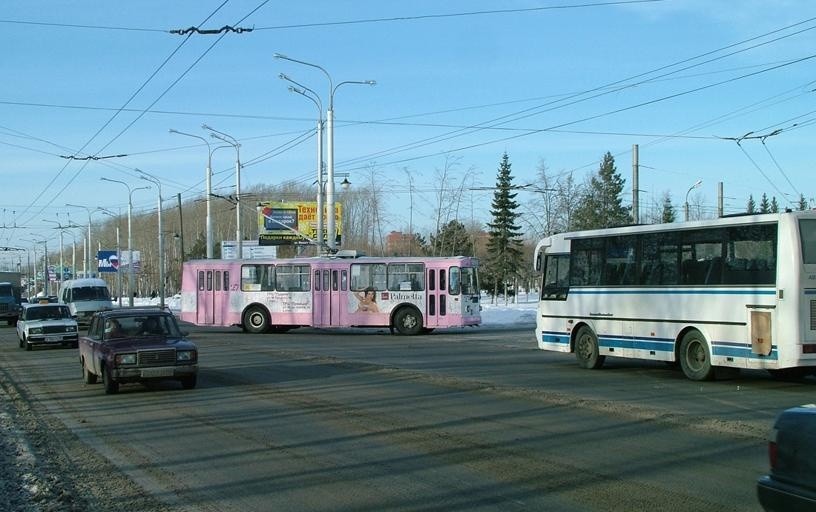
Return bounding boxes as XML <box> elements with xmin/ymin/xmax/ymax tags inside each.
<box><xmin>0</xmin><ymin>271</ymin><xmax>23</xmax><ymax>326</ymax></box>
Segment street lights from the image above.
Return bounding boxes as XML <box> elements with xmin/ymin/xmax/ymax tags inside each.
<box><xmin>19</xmin><ymin>205</ymin><xmax>121</xmax><ymax>308</ymax></box>
<box><xmin>273</xmin><ymin>53</ymin><xmax>376</xmax><ymax>256</ymax></box>
<box><xmin>169</xmin><ymin>123</ymin><xmax>242</xmax><ymax>258</ymax></box>
<box><xmin>101</xmin><ymin>168</ymin><xmax>165</xmax><ymax>310</ymax></box>
<box><xmin>684</xmin><ymin>180</ymin><xmax>703</xmax><ymax>221</ymax></box>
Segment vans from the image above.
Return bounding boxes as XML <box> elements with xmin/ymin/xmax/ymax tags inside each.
<box><xmin>58</xmin><ymin>279</ymin><xmax>113</xmax><ymax>330</ymax></box>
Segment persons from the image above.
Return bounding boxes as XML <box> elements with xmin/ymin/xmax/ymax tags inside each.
<box><xmin>104</xmin><ymin>320</ymin><xmax>125</xmax><ymax>339</ymax></box>
<box><xmin>353</xmin><ymin>286</ymin><xmax>379</xmax><ymax>313</ymax></box>
<box><xmin>135</xmin><ymin>320</ymin><xmax>160</xmax><ymax>336</ymax></box>
<box><xmin>150</xmin><ymin>291</ymin><xmax>157</xmax><ymax>300</ymax></box>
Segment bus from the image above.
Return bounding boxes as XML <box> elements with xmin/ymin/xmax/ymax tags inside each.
<box><xmin>179</xmin><ymin>256</ymin><xmax>482</xmax><ymax>335</ymax></box>
<box><xmin>533</xmin><ymin>206</ymin><xmax>816</xmax><ymax>381</ymax></box>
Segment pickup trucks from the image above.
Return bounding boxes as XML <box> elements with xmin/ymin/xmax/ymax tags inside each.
<box><xmin>79</xmin><ymin>308</ymin><xmax>198</xmax><ymax>393</ymax></box>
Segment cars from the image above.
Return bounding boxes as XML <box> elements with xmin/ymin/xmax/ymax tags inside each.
<box><xmin>17</xmin><ymin>300</ymin><xmax>78</xmax><ymax>351</ymax></box>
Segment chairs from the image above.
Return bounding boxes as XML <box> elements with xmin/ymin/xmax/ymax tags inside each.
<box><xmin>601</xmin><ymin>256</ymin><xmax>767</xmax><ymax>284</ymax></box>
